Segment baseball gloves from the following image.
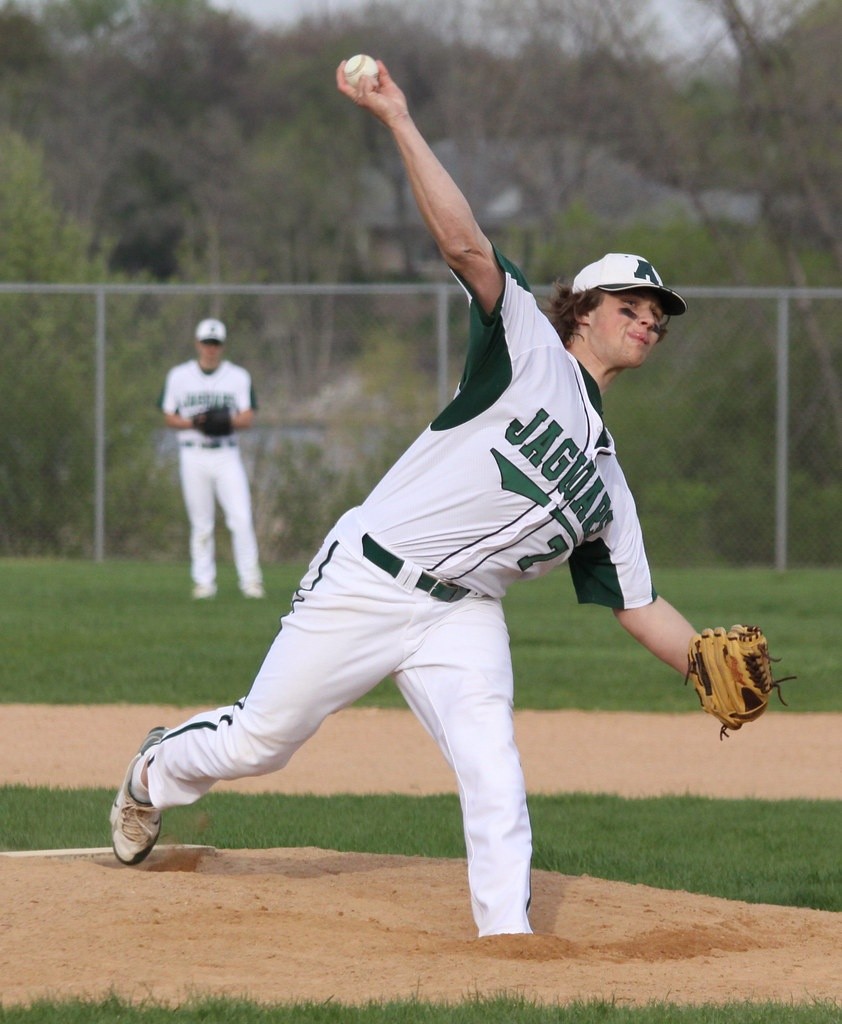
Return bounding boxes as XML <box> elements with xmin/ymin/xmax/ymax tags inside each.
<box><xmin>190</xmin><ymin>402</ymin><xmax>234</xmax><ymax>439</ymax></box>
<box><xmin>685</xmin><ymin>624</ymin><xmax>774</xmax><ymax>726</ymax></box>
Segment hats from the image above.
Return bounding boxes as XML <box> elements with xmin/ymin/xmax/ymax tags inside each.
<box><xmin>571</xmin><ymin>253</ymin><xmax>688</xmax><ymax>316</ymax></box>
<box><xmin>196</xmin><ymin>318</ymin><xmax>227</xmax><ymax>343</ymax></box>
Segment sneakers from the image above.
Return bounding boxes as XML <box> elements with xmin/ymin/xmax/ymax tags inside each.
<box><xmin>109</xmin><ymin>726</ymin><xmax>176</xmax><ymax>866</ymax></box>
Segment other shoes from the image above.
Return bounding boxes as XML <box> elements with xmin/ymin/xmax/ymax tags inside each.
<box><xmin>191</xmin><ymin>581</ymin><xmax>218</xmax><ymax>600</ymax></box>
<box><xmin>240</xmin><ymin>581</ymin><xmax>264</xmax><ymax>598</ymax></box>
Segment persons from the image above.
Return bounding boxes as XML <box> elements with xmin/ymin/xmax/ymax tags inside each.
<box><xmin>162</xmin><ymin>320</ymin><xmax>267</xmax><ymax>600</ymax></box>
<box><xmin>109</xmin><ymin>60</ymin><xmax>772</xmax><ymax>936</ymax></box>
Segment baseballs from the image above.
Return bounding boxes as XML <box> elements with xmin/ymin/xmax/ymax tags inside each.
<box><xmin>344</xmin><ymin>54</ymin><xmax>379</xmax><ymax>89</ymax></box>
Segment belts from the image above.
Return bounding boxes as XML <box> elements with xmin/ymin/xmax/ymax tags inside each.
<box><xmin>362</xmin><ymin>533</ymin><xmax>470</xmax><ymax>603</ymax></box>
<box><xmin>185</xmin><ymin>441</ymin><xmax>237</xmax><ymax>448</ymax></box>
